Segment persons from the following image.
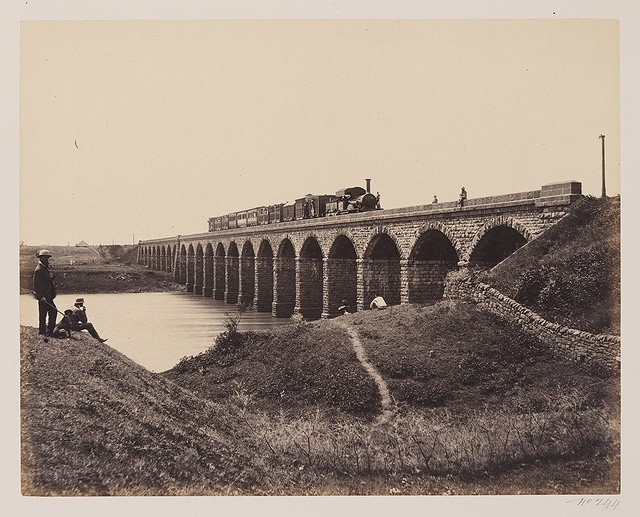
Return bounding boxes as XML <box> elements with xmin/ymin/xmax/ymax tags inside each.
<box><xmin>33</xmin><ymin>249</ymin><xmax>57</xmax><ymax>334</ymax></box>
<box><xmin>338</xmin><ymin>300</ymin><xmax>350</xmax><ymax>314</ymax></box>
<box><xmin>68</xmin><ymin>297</ymin><xmax>108</xmax><ymax>342</ymax></box>
<box><xmin>459</xmin><ymin>185</ymin><xmax>468</xmax><ymax>207</ymax></box>
<box><xmin>369</xmin><ymin>293</ymin><xmax>387</xmax><ymax>310</ymax></box>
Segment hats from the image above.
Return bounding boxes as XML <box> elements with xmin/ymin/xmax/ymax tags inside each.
<box><xmin>75</xmin><ymin>298</ymin><xmax>85</xmax><ymax>304</ymax></box>
<box><xmin>37</xmin><ymin>250</ymin><xmax>52</xmax><ymax>258</ymax></box>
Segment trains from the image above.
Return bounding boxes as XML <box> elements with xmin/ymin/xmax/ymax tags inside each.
<box><xmin>207</xmin><ymin>178</ymin><xmax>384</xmax><ymax>232</ymax></box>
